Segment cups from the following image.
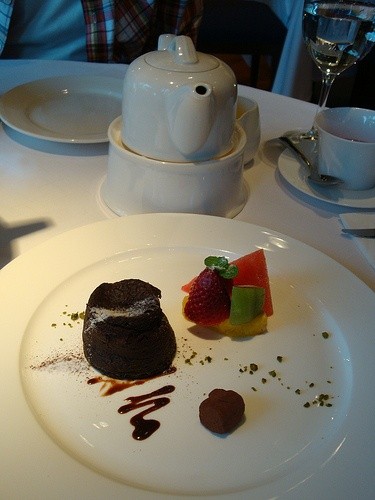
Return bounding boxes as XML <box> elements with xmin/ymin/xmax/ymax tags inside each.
<box><xmin>314</xmin><ymin>106</ymin><xmax>375</xmax><ymax>191</ymax></box>
<box><xmin>236</xmin><ymin>97</ymin><xmax>261</xmax><ymax>164</ymax></box>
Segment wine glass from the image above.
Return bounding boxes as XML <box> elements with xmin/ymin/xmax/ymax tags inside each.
<box><xmin>301</xmin><ymin>0</ymin><xmax>375</xmax><ymax>132</ymax></box>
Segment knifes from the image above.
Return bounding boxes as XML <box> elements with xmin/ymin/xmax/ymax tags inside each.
<box><xmin>341</xmin><ymin>228</ymin><xmax>375</xmax><ymax>238</ymax></box>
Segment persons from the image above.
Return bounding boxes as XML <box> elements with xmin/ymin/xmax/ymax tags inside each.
<box><xmin>0</xmin><ymin>0</ymin><xmax>278</xmax><ymax>92</ymax></box>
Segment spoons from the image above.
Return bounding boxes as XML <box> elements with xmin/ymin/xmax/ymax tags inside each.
<box><xmin>280</xmin><ymin>136</ymin><xmax>344</xmax><ymax>187</ymax></box>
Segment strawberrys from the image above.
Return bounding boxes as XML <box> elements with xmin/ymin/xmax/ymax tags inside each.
<box><xmin>183</xmin><ymin>256</ymin><xmax>238</xmax><ymax>326</ymax></box>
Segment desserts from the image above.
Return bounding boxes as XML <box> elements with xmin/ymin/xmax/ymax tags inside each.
<box><xmin>82</xmin><ymin>279</ymin><xmax>176</xmax><ymax>379</ymax></box>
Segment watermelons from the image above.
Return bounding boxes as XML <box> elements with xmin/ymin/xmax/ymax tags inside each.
<box><xmin>181</xmin><ymin>249</ymin><xmax>273</xmax><ymax>317</ymax></box>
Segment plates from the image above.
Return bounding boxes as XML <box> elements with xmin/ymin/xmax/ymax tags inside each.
<box><xmin>1</xmin><ymin>212</ymin><xmax>375</xmax><ymax>500</ymax></box>
<box><xmin>277</xmin><ymin>139</ymin><xmax>375</xmax><ymax>209</ymax></box>
<box><xmin>0</xmin><ymin>75</ymin><xmax>121</xmax><ymax>143</ymax></box>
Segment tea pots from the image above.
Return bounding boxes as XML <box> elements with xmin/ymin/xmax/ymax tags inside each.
<box><xmin>121</xmin><ymin>33</ymin><xmax>240</xmax><ymax>162</ymax></box>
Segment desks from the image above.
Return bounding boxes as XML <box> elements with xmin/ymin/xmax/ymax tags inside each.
<box><xmin>0</xmin><ymin>62</ymin><xmax>374</xmax><ymax>289</ymax></box>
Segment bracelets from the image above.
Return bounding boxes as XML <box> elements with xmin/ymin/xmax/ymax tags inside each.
<box><xmin>251</xmin><ymin>55</ymin><xmax>260</xmax><ymax>87</ymax></box>
